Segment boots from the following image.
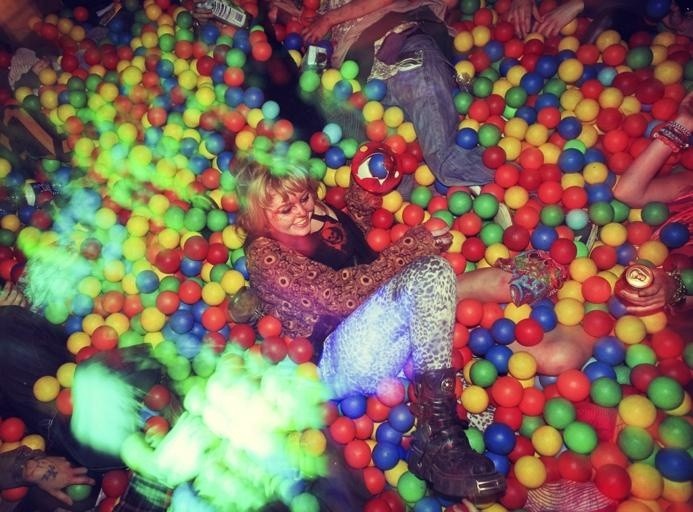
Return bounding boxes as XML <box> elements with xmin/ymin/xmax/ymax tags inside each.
<box><xmin>405</xmin><ymin>367</ymin><xmax>505</xmax><ymax>499</ymax></box>
